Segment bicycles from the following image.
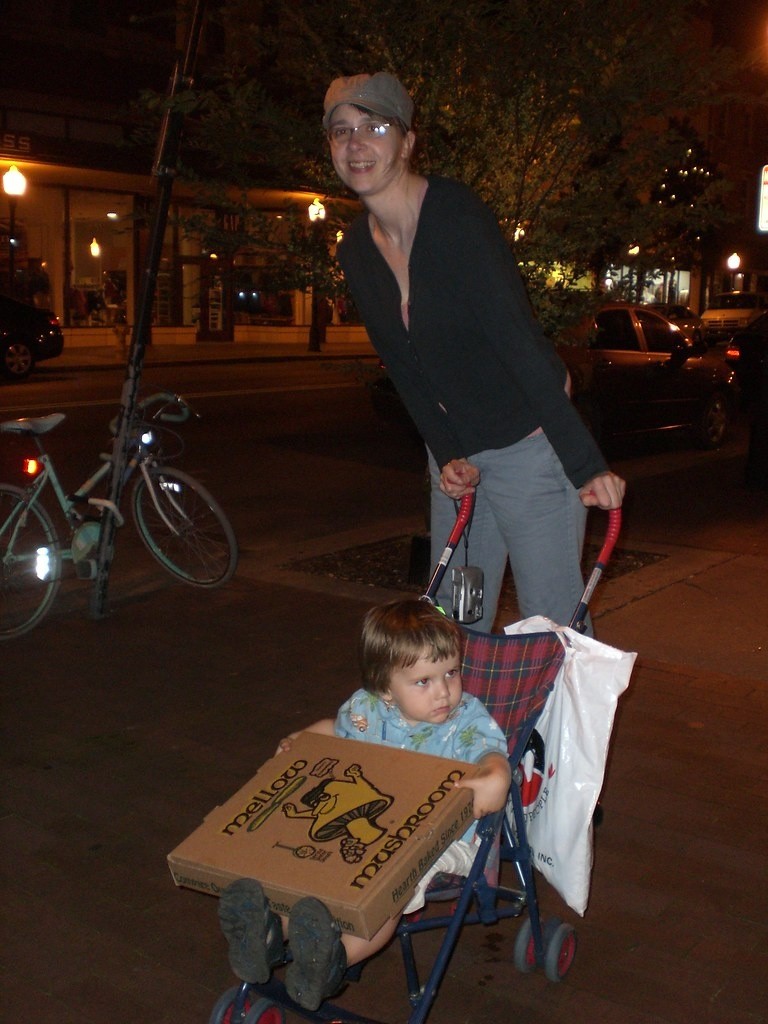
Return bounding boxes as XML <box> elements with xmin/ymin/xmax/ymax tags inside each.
<box><xmin>0</xmin><ymin>392</ymin><xmax>240</xmax><ymax>643</ymax></box>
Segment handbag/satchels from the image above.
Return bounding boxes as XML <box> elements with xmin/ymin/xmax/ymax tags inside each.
<box><xmin>504</xmin><ymin>615</ymin><xmax>638</xmax><ymax>917</ymax></box>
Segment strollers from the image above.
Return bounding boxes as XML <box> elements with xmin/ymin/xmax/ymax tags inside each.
<box><xmin>207</xmin><ymin>472</ymin><xmax>623</xmax><ymax>1024</ymax></box>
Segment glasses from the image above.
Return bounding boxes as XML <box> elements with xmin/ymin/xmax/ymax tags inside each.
<box><xmin>325</xmin><ymin>120</ymin><xmax>394</xmax><ymax>142</ymax></box>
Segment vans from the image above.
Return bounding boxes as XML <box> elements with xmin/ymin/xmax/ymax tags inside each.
<box><xmin>700</xmin><ymin>290</ymin><xmax>768</xmax><ymax>341</ymax></box>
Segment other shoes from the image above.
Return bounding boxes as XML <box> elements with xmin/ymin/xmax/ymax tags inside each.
<box><xmin>217</xmin><ymin>878</ymin><xmax>289</xmax><ymax>984</ymax></box>
<box><xmin>285</xmin><ymin>896</ymin><xmax>347</xmax><ymax>1011</ymax></box>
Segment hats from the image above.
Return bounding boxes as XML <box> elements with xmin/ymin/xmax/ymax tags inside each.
<box><xmin>322</xmin><ymin>71</ymin><xmax>414</xmax><ymax>130</ymax></box>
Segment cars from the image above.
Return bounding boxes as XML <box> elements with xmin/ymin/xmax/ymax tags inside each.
<box><xmin>0</xmin><ymin>296</ymin><xmax>66</xmax><ymax>379</ymax></box>
<box><xmin>635</xmin><ymin>300</ymin><xmax>709</xmax><ymax>355</ymax></box>
<box><xmin>725</xmin><ymin>311</ymin><xmax>768</xmax><ymax>399</ymax></box>
<box><xmin>372</xmin><ymin>300</ymin><xmax>742</xmax><ymax>451</ymax></box>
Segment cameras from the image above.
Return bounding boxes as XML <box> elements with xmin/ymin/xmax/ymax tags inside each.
<box><xmin>451</xmin><ymin>567</ymin><xmax>484</xmax><ymax>625</ymax></box>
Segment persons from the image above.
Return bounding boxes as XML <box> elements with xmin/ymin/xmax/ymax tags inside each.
<box><xmin>320</xmin><ymin>71</ymin><xmax>626</xmax><ymax>826</ymax></box>
<box><xmin>218</xmin><ymin>599</ymin><xmax>513</xmax><ymax>1006</ymax></box>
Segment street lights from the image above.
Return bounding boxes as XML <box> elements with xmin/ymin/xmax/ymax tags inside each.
<box><xmin>725</xmin><ymin>252</ymin><xmax>742</xmax><ymax>292</ymax></box>
<box><xmin>2</xmin><ymin>164</ymin><xmax>29</xmax><ymax>301</ymax></box>
<box><xmin>90</xmin><ymin>237</ymin><xmax>106</xmax><ymax>310</ymax></box>
<box><xmin>308</xmin><ymin>200</ymin><xmax>327</xmax><ymax>353</ymax></box>
